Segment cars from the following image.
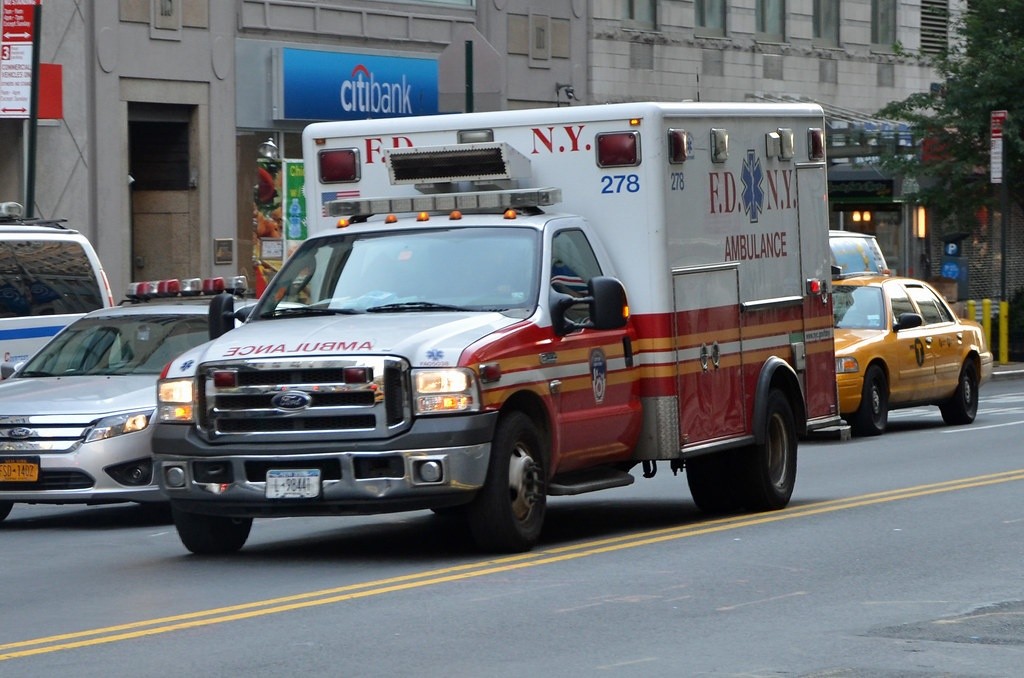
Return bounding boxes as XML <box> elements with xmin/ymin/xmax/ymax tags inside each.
<box><xmin>0</xmin><ymin>276</ymin><xmax>306</xmax><ymax>524</ymax></box>
<box><xmin>825</xmin><ymin>266</ymin><xmax>996</xmax><ymax>436</ymax></box>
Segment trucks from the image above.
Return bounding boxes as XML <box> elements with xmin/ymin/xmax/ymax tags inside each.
<box><xmin>151</xmin><ymin>101</ymin><xmax>836</xmax><ymax>562</ymax></box>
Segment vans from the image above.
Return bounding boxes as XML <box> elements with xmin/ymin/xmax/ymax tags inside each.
<box><xmin>1</xmin><ymin>202</ymin><xmax>116</xmax><ymax>387</ymax></box>
